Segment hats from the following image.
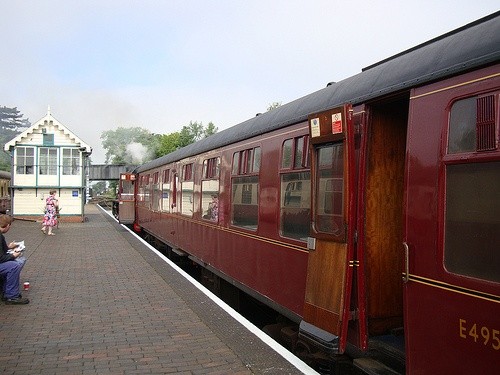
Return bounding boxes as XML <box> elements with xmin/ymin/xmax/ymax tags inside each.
<box><xmin>0</xmin><ymin>214</ymin><xmax>12</xmax><ymax>227</ymax></box>
<box><xmin>50</xmin><ymin>190</ymin><xmax>56</xmax><ymax>194</ymax></box>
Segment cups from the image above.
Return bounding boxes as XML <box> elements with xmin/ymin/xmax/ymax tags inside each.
<box><xmin>24</xmin><ymin>282</ymin><xmax>30</xmax><ymax>290</ymax></box>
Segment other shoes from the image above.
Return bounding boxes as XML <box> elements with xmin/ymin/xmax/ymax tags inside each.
<box><xmin>48</xmin><ymin>232</ymin><xmax>55</xmax><ymax>236</ymax></box>
<box><xmin>2</xmin><ymin>294</ymin><xmax>22</xmax><ymax>301</ymax></box>
<box><xmin>41</xmin><ymin>229</ymin><xmax>46</xmax><ymax>235</ymax></box>
<box><xmin>5</xmin><ymin>298</ymin><xmax>29</xmax><ymax>305</ymax></box>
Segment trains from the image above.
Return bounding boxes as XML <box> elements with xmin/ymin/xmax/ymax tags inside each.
<box><xmin>112</xmin><ymin>10</ymin><xmax>500</xmax><ymax>375</ymax></box>
<box><xmin>0</xmin><ymin>171</ymin><xmax>12</xmax><ymax>213</ymax></box>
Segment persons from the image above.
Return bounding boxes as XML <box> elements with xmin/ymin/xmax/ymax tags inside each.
<box><xmin>0</xmin><ymin>212</ymin><xmax>30</xmax><ymax>305</ymax></box>
<box><xmin>41</xmin><ymin>189</ymin><xmax>60</xmax><ymax>236</ymax></box>
<box><xmin>208</xmin><ymin>193</ymin><xmax>218</xmax><ymax>222</ymax></box>
